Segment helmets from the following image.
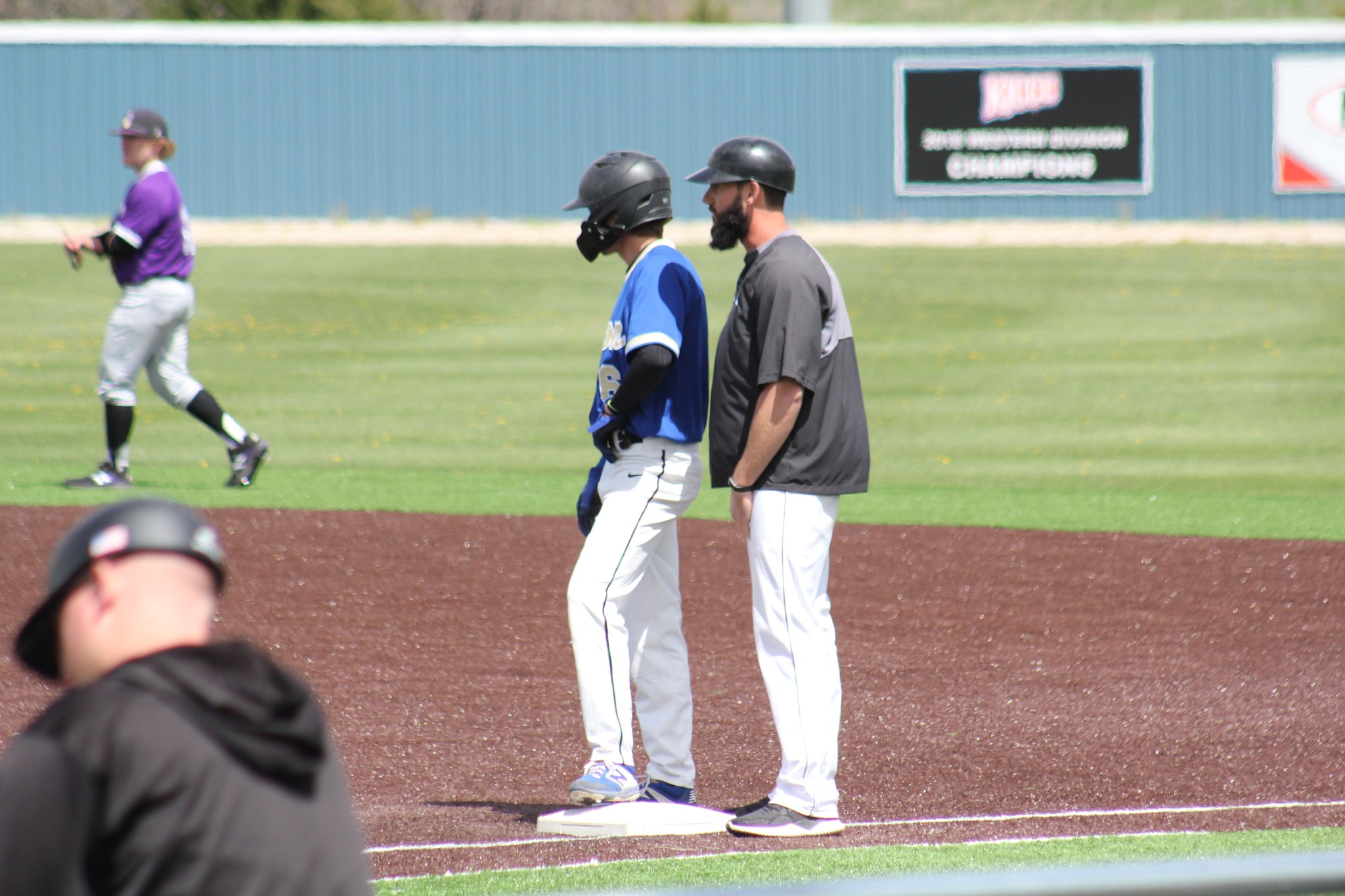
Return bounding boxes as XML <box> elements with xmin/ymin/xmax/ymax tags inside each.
<box><xmin>14</xmin><ymin>500</ymin><xmax>224</xmax><ymax>678</ymax></box>
<box><xmin>562</xmin><ymin>151</ymin><xmax>672</xmax><ymax>262</ymax></box>
<box><xmin>683</xmin><ymin>137</ymin><xmax>795</xmax><ymax>194</ymax></box>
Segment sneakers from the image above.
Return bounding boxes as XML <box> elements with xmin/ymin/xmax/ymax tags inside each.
<box><xmin>636</xmin><ymin>774</ymin><xmax>697</xmax><ymax>805</ymax></box>
<box><xmin>226</xmin><ymin>433</ymin><xmax>266</xmax><ymax>486</ymax></box>
<box><xmin>728</xmin><ymin>804</ymin><xmax>845</xmax><ymax>837</ymax></box>
<box><xmin>724</xmin><ymin>796</ymin><xmax>770</xmax><ymax>817</ymax></box>
<box><xmin>66</xmin><ymin>464</ymin><xmax>133</xmax><ymax>490</ymax></box>
<box><xmin>568</xmin><ymin>760</ymin><xmax>639</xmax><ymax>805</ymax></box>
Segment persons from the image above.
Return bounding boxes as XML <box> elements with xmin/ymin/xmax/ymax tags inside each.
<box><xmin>63</xmin><ymin>109</ymin><xmax>269</xmax><ymax>492</ymax></box>
<box><xmin>683</xmin><ymin>135</ymin><xmax>871</xmax><ymax>837</ymax></box>
<box><xmin>0</xmin><ymin>496</ymin><xmax>371</xmax><ymax>895</ymax></box>
<box><xmin>556</xmin><ymin>151</ymin><xmax>711</xmax><ymax>805</ymax></box>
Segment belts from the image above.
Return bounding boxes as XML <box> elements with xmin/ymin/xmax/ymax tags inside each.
<box><xmin>618</xmin><ymin>430</ymin><xmax>642</xmax><ymax>443</ymax></box>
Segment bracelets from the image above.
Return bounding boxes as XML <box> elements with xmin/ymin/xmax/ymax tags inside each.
<box><xmin>727</xmin><ymin>476</ymin><xmax>754</xmax><ymax>492</ymax></box>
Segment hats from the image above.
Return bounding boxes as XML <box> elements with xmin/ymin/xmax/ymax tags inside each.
<box><xmin>109</xmin><ymin>110</ymin><xmax>168</xmax><ymax>138</ymax></box>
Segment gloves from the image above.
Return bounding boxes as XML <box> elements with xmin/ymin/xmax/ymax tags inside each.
<box><xmin>588</xmin><ymin>397</ymin><xmax>625</xmax><ymax>463</ymax></box>
<box><xmin>576</xmin><ymin>467</ymin><xmax>601</xmax><ymax>537</ymax></box>
